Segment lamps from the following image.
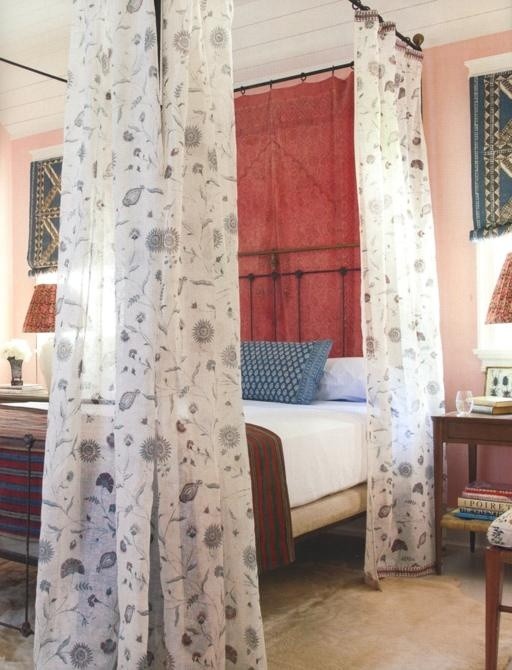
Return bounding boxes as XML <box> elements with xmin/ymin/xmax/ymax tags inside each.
<box><xmin>23</xmin><ymin>283</ymin><xmax>56</xmax><ymax>333</ymax></box>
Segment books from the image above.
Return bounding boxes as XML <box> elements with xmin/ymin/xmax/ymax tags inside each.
<box><xmin>450</xmin><ymin>482</ymin><xmax>512</xmax><ymax>521</ymax></box>
<box><xmin>466</xmin><ymin>395</ymin><xmax>512</xmax><ymax>408</ymax></box>
<box><xmin>470</xmin><ymin>405</ymin><xmax>512</xmax><ymax>414</ymax></box>
<box><xmin>0</xmin><ymin>384</ymin><xmax>46</xmax><ymax>397</ymax></box>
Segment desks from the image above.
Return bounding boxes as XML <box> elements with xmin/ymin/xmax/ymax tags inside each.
<box><xmin>431</xmin><ymin>405</ymin><xmax>511</xmax><ymax>575</ymax></box>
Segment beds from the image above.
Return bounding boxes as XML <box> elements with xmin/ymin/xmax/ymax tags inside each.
<box><xmin>0</xmin><ymin>400</ymin><xmax>386</xmax><ymax>635</ymax></box>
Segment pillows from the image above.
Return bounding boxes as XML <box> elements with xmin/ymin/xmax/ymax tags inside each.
<box><xmin>238</xmin><ymin>340</ymin><xmax>368</xmax><ymax>405</ymax></box>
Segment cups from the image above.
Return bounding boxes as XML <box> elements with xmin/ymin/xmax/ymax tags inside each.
<box><xmin>455</xmin><ymin>390</ymin><xmax>474</xmax><ymax>417</ymax></box>
